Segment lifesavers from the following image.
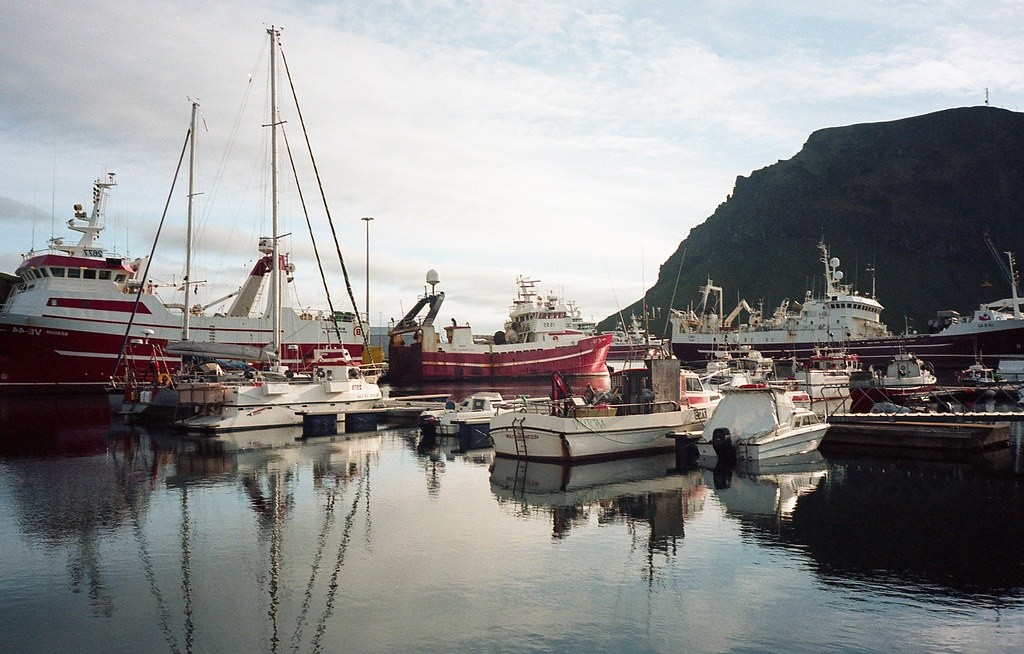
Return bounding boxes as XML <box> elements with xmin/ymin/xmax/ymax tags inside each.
<box><xmin>158</xmin><ymin>373</ymin><xmax>171</xmax><ymax>384</ymax></box>
<box><xmin>553</xmin><ymin>336</ymin><xmax>558</xmax><ymax>340</ymax></box>
<box><xmin>785</xmin><ymin>377</ymin><xmax>799</xmax><ymax>391</ymax></box>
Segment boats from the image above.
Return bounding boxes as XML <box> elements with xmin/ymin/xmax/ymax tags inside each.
<box><xmin>0</xmin><ymin>20</ymin><xmax>1024</xmax><ymax>462</ymax></box>
